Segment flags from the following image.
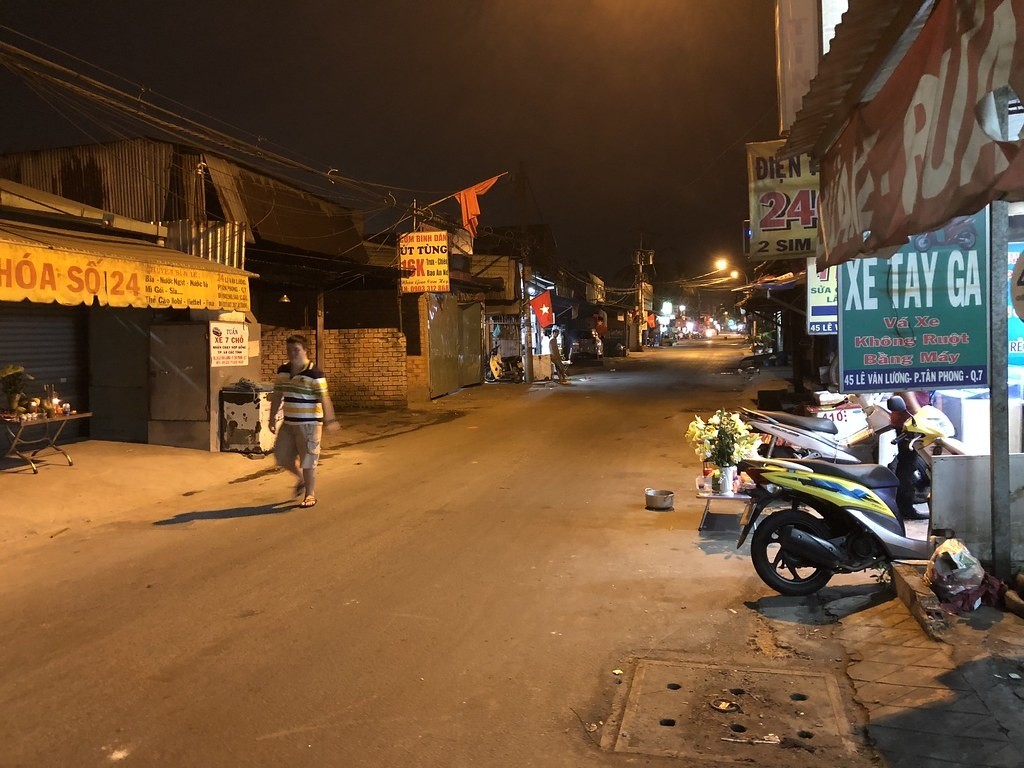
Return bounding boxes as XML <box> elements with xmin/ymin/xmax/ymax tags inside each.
<box><xmin>596</xmin><ymin>320</ymin><xmax>608</xmax><ymax>335</ymax></box>
<box><xmin>455</xmin><ymin>177</ymin><xmax>498</xmax><ymax>237</ymax></box>
<box><xmin>530</xmin><ymin>290</ymin><xmax>553</xmax><ymax>328</ymax></box>
<box><xmin>647</xmin><ymin>314</ymin><xmax>655</xmax><ymax>328</ymax></box>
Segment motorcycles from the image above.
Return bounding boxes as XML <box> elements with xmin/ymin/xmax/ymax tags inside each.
<box><xmin>484</xmin><ymin>346</ymin><xmax>524</xmax><ymax>384</ymax></box>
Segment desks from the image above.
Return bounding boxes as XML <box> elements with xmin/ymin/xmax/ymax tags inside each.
<box><xmin>0</xmin><ymin>411</ymin><xmax>93</xmax><ymax>474</ymax></box>
<box><xmin>695</xmin><ymin>494</ymin><xmax>757</xmax><ymax>532</ymax></box>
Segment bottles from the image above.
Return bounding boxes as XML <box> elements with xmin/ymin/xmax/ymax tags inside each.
<box><xmin>63</xmin><ymin>402</ymin><xmax>70</xmax><ymax>416</ymax></box>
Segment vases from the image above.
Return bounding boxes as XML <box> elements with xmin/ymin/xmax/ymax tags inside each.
<box><xmin>9</xmin><ymin>398</ymin><xmax>18</xmax><ymax>409</ymax></box>
<box><xmin>718</xmin><ymin>467</ymin><xmax>735</xmax><ymax>496</ymax></box>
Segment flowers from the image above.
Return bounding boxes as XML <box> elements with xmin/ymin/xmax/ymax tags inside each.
<box><xmin>0</xmin><ymin>364</ymin><xmax>34</xmax><ymax>395</ymax></box>
<box><xmin>684</xmin><ymin>410</ymin><xmax>763</xmax><ymax>464</ymax></box>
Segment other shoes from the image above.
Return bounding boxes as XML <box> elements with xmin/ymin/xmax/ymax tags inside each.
<box><xmin>900</xmin><ymin>508</ymin><xmax>930</xmax><ymax>519</ymax></box>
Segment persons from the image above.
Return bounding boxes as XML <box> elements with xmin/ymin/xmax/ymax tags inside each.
<box><xmin>269</xmin><ymin>335</ymin><xmax>340</xmax><ymax>507</ymax></box>
<box><xmin>549</xmin><ymin>329</ymin><xmax>567</xmax><ymax>383</ymax></box>
<box><xmin>889</xmin><ymin>390</ymin><xmax>930</xmax><ymax>519</ymax></box>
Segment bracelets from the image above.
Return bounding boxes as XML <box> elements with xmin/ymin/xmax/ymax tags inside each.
<box><xmin>325</xmin><ymin>418</ymin><xmax>337</xmax><ymax>426</ymax></box>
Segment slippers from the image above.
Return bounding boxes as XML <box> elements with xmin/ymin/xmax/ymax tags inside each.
<box><xmin>300</xmin><ymin>498</ymin><xmax>317</xmax><ymax>507</ymax></box>
<box><xmin>297</xmin><ymin>481</ymin><xmax>305</xmax><ymax>495</ymax></box>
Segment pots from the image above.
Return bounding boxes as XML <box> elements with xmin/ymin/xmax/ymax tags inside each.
<box><xmin>644</xmin><ymin>488</ymin><xmax>674</xmax><ymax>509</ymax></box>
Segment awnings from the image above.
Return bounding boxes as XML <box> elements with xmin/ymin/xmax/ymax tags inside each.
<box><xmin>0</xmin><ymin>225</ymin><xmax>260</xmax><ymax>312</ymax></box>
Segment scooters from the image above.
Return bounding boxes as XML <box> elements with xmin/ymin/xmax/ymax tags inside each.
<box><xmin>735</xmin><ymin>392</ymin><xmax>931</xmax><ymax>528</ymax></box>
<box><xmin>732</xmin><ymin>396</ymin><xmax>976</xmax><ymax>598</ymax></box>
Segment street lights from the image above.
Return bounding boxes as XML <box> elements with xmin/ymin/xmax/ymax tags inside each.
<box><xmin>715</xmin><ymin>259</ymin><xmax>751</xmax><ymax>341</ymax></box>
